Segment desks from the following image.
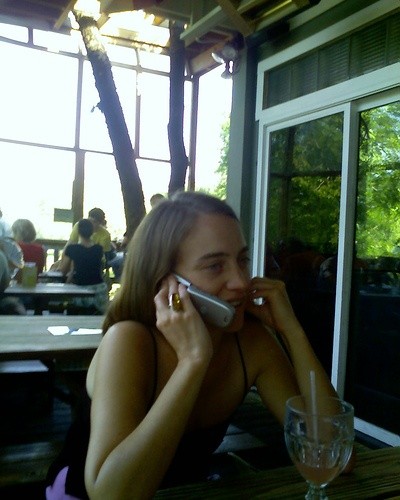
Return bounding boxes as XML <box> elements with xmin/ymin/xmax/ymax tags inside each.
<box><xmin>5</xmin><ymin>281</ymin><xmax>100</xmax><ymax>314</ymax></box>
<box><xmin>1</xmin><ymin>313</ymin><xmax>108</xmax><ymax>360</ymax></box>
<box><xmin>149</xmin><ymin>446</ymin><xmax>400</xmax><ymax>499</ymax></box>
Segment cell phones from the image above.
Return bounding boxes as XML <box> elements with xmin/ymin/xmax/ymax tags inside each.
<box><xmin>168</xmin><ymin>273</ymin><xmax>234</xmax><ymax>333</ymax></box>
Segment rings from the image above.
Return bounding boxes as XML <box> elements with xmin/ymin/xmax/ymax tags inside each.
<box><xmin>167</xmin><ymin>292</ymin><xmax>183</xmax><ymax>313</ymax></box>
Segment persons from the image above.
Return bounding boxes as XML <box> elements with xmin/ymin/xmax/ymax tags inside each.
<box><xmin>61</xmin><ymin>219</ymin><xmax>103</xmax><ymax>285</ymax></box>
<box><xmin>45</xmin><ymin>191</ymin><xmax>338</xmax><ymax>500</ymax></box>
<box><xmin>65</xmin><ymin>207</ymin><xmax>112</xmax><ymax>266</ymax></box>
<box><xmin>149</xmin><ymin>193</ymin><xmax>166</xmax><ymax>209</ymax></box>
<box><xmin>5</xmin><ymin>219</ymin><xmax>46</xmax><ymax>276</ymax></box>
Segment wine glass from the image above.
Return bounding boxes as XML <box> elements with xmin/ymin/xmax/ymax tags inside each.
<box><xmin>283</xmin><ymin>395</ymin><xmax>356</xmax><ymax>499</ymax></box>
